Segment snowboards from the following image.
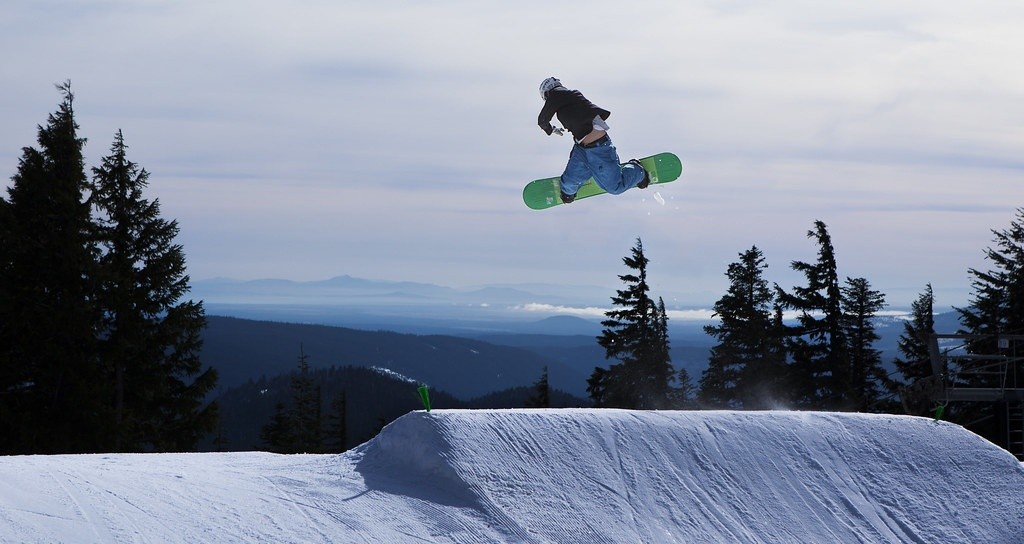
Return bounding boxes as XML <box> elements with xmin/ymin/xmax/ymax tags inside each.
<box><xmin>524</xmin><ymin>152</ymin><xmax>683</xmax><ymax>212</ymax></box>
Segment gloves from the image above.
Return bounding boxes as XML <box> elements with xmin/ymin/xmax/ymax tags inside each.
<box><xmin>552</xmin><ymin>126</ymin><xmax>564</xmax><ymax>136</ymax></box>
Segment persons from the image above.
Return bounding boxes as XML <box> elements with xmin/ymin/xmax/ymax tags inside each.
<box><xmin>537</xmin><ymin>76</ymin><xmax>650</xmax><ymax>204</ymax></box>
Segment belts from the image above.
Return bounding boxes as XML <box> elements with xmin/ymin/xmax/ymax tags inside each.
<box><xmin>576</xmin><ymin>133</ymin><xmax>609</xmax><ymax>149</ymax></box>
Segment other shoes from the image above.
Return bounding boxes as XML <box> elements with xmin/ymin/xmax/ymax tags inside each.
<box><xmin>560</xmin><ymin>190</ymin><xmax>576</xmax><ymax>203</ymax></box>
<box><xmin>628</xmin><ymin>159</ymin><xmax>650</xmax><ymax>189</ymax></box>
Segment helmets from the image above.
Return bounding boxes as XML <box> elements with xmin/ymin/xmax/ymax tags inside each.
<box><xmin>539</xmin><ymin>77</ymin><xmax>562</xmax><ymax>102</ymax></box>
